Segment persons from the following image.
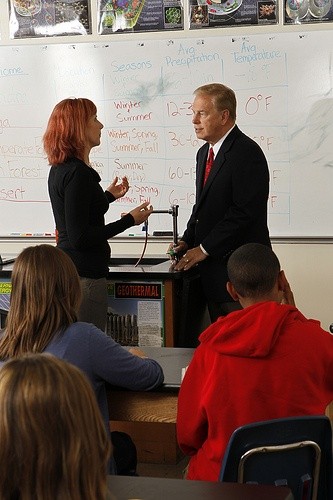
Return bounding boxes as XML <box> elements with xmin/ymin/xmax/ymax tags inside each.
<box><xmin>41</xmin><ymin>97</ymin><xmax>153</xmax><ymax>334</ymax></box>
<box><xmin>176</xmin><ymin>243</ymin><xmax>332</xmax><ymax>482</ymax></box>
<box><xmin>166</xmin><ymin>83</ymin><xmax>273</xmax><ymax>324</ymax></box>
<box><xmin>0</xmin><ymin>244</ymin><xmax>166</xmax><ymax>500</ymax></box>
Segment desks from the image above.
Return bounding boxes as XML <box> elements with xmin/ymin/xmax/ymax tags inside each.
<box><xmin>0</xmin><ymin>254</ymin><xmax>184</xmax><ymax>347</ymax></box>
<box><xmin>107</xmin><ymin>347</ymin><xmax>196</xmax><ymax>423</ymax></box>
<box><xmin>106</xmin><ymin>475</ymin><xmax>293</xmax><ymax>500</ymax></box>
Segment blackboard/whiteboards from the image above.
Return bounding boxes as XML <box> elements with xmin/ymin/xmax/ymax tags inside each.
<box><xmin>0</xmin><ymin>23</ymin><xmax>333</xmax><ymax>244</ymax></box>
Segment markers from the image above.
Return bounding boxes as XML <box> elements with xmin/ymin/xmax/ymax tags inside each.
<box><xmin>128</xmin><ymin>234</ymin><xmax>148</xmax><ymax>236</ymax></box>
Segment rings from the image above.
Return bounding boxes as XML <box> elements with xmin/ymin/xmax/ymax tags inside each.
<box><xmin>186</xmin><ymin>259</ymin><xmax>189</xmax><ymax>262</ymax></box>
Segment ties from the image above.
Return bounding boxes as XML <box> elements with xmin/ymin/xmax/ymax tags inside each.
<box><xmin>202</xmin><ymin>148</ymin><xmax>213</xmax><ymax>190</ymax></box>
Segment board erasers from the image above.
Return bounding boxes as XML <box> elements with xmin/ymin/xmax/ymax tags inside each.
<box><xmin>152</xmin><ymin>231</ymin><xmax>172</xmax><ymax>236</ymax></box>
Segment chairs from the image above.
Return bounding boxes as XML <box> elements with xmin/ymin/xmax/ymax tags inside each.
<box><xmin>221</xmin><ymin>415</ymin><xmax>333</xmax><ymax>500</ymax></box>
